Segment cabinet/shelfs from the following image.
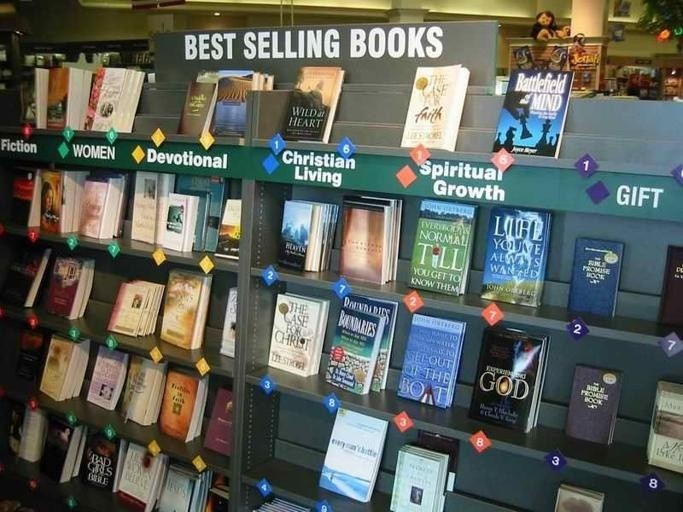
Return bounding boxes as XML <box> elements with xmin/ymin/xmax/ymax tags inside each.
<box><xmin>0</xmin><ymin>91</ymin><xmax>683</xmax><ymax>510</ymax></box>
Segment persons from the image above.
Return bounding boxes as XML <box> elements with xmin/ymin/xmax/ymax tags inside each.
<box><xmin>532</xmin><ymin>10</ymin><xmax>557</xmax><ymax>42</ymax></box>
